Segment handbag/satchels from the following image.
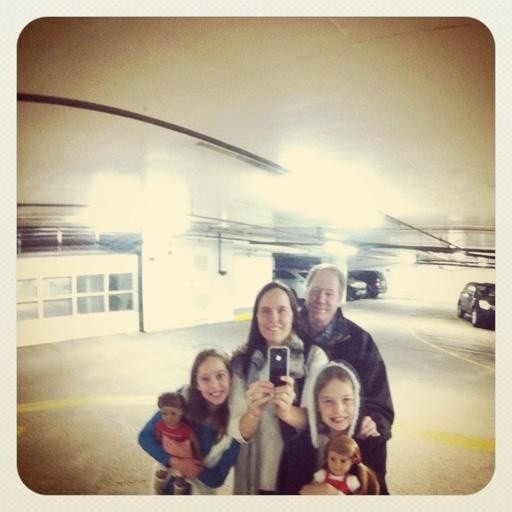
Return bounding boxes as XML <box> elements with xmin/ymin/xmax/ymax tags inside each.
<box><xmin>275</xmin><ymin>430</ymin><xmax>317</xmax><ymax>495</ymax></box>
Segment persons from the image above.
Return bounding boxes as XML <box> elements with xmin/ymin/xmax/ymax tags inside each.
<box><xmin>153</xmin><ymin>392</ymin><xmax>204</xmax><ymax>494</ymax></box>
<box><xmin>231</xmin><ymin>263</ymin><xmax>394</xmax><ymax>495</ymax></box>
<box><xmin>137</xmin><ymin>348</ymin><xmax>241</xmax><ymax>495</ymax></box>
<box><xmin>273</xmin><ymin>360</ymin><xmax>390</xmax><ymax>495</ymax></box>
<box><xmin>225</xmin><ymin>280</ymin><xmax>329</xmax><ymax>495</ymax></box>
<box><xmin>313</xmin><ymin>435</ymin><xmax>380</xmax><ymax>496</ymax></box>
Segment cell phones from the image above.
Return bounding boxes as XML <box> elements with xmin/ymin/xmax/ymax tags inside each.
<box><xmin>267</xmin><ymin>345</ymin><xmax>289</xmax><ymax>386</ymax></box>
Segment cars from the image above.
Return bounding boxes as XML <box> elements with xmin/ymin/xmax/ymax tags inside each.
<box><xmin>271</xmin><ymin>267</ymin><xmax>387</xmax><ymax>309</ymax></box>
<box><xmin>458</xmin><ymin>281</ymin><xmax>496</xmax><ymax>329</ymax></box>
